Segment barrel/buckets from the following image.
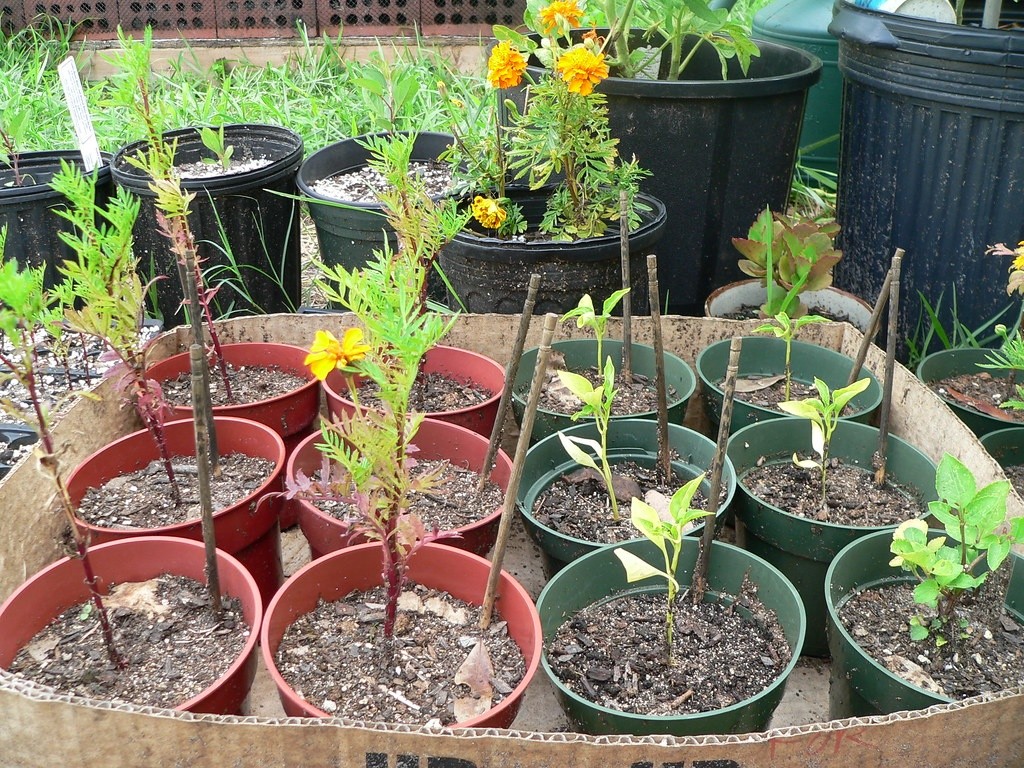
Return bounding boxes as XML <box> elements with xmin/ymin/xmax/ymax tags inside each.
<box><xmin>827</xmin><ymin>0</ymin><xmax>1024</xmax><ymax>376</ymax></box>
<box><xmin>852</xmin><ymin>0</ymin><xmax>956</xmax><ymax>30</ymax></box>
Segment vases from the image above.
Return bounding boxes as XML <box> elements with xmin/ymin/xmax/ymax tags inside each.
<box><xmin>832</xmin><ymin>0</ymin><xmax>1023</xmax><ymax>364</ymax></box>
<box><xmin>915</xmin><ymin>348</ymin><xmax>1023</xmax><ymax>438</ymax></box>
<box><xmin>426</xmin><ymin>179</ymin><xmax>669</xmax><ymax>321</ymax></box>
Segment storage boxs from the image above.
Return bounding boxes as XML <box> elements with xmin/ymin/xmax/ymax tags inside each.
<box><xmin>112</xmin><ymin>96</ymin><xmax>1024</xmax><ymax>768</ymax></box>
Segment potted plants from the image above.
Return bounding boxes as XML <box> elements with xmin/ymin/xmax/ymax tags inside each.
<box><xmin>284</xmin><ymin>47</ymin><xmax>516</xmax><ymax>562</ymax></box>
<box><xmin>715</xmin><ymin>375</ymin><xmax>946</xmax><ymax>661</ymax></box>
<box><xmin>106</xmin><ymin>80</ymin><xmax>307</xmax><ymax>336</ymax></box>
<box><xmin>513</xmin><ymin>355</ymin><xmax>737</xmax><ymax>582</ymax></box>
<box><xmin>535</xmin><ymin>467</ymin><xmax>807</xmax><ymax>738</ymax></box>
<box><xmin>823</xmin><ymin>448</ymin><xmax>1024</xmax><ymax>728</ymax></box>
<box><xmin>259</xmin><ymin>540</ymin><xmax>542</xmax><ymax>730</ymax></box>
<box><xmin>702</xmin><ymin>205</ymin><xmax>882</xmax><ymax>347</ymax></box>
<box><xmin>506</xmin><ymin>285</ymin><xmax>696</xmax><ymax>450</ymax></box>
<box><xmin>498</xmin><ymin>0</ymin><xmax>825</xmax><ymax>316</ymax></box>
<box><xmin>693</xmin><ymin>313</ymin><xmax>884</xmax><ymax>452</ymax></box>
<box><xmin>0</xmin><ymin>220</ymin><xmax>261</xmax><ymax>717</ymax></box>
<box><xmin>78</xmin><ymin>19</ymin><xmax>320</xmax><ymax>460</ymax></box>
<box><xmin>57</xmin><ymin>154</ymin><xmax>287</xmax><ymax>623</ymax></box>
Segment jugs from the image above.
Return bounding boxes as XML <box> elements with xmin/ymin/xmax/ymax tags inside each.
<box><xmin>703</xmin><ymin>0</ymin><xmax>852</xmax><ymax>190</ymax></box>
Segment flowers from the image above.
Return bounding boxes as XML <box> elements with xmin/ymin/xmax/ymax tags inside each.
<box><xmin>467</xmin><ymin>0</ymin><xmax>653</xmax><ymax>240</ymax></box>
<box><xmin>254</xmin><ymin>235</ymin><xmax>460</xmax><ymax>642</ymax></box>
<box><xmin>981</xmin><ymin>238</ymin><xmax>1024</xmax><ymax>397</ymax></box>
